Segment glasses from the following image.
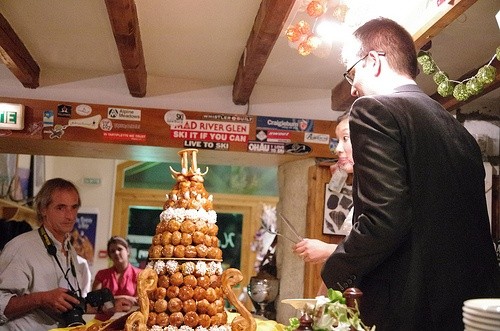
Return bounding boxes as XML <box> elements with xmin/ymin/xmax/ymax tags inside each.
<box><xmin>343</xmin><ymin>51</ymin><xmax>385</xmax><ymax>85</ymax></box>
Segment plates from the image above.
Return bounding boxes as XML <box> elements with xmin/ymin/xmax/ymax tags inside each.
<box><xmin>462</xmin><ymin>298</ymin><xmax>500</xmax><ymax>331</ymax></box>
<box><xmin>281</xmin><ymin>299</ymin><xmax>329</xmax><ymax>310</ymax></box>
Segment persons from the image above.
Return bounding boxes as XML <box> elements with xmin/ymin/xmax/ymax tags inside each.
<box><xmin>0</xmin><ymin>178</ymin><xmax>142</xmax><ymax>331</ymax></box>
<box><xmin>292</xmin><ymin>17</ymin><xmax>500</xmax><ymax>331</ymax></box>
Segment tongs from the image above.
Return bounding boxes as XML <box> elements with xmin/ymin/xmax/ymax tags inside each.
<box><xmin>260</xmin><ymin>213</ymin><xmax>301</xmax><ymax>245</ymax></box>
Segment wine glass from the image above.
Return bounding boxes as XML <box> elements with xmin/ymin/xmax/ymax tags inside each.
<box><xmin>249</xmin><ymin>276</ymin><xmax>280</xmax><ymax>317</ymax></box>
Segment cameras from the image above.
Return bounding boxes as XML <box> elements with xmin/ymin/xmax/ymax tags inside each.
<box><xmin>55</xmin><ymin>288</ymin><xmax>114</xmax><ymax>329</ymax></box>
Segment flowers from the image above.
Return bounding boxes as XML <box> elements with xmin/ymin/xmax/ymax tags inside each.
<box><xmin>275</xmin><ymin>288</ymin><xmax>374</xmax><ymax>331</ymax></box>
